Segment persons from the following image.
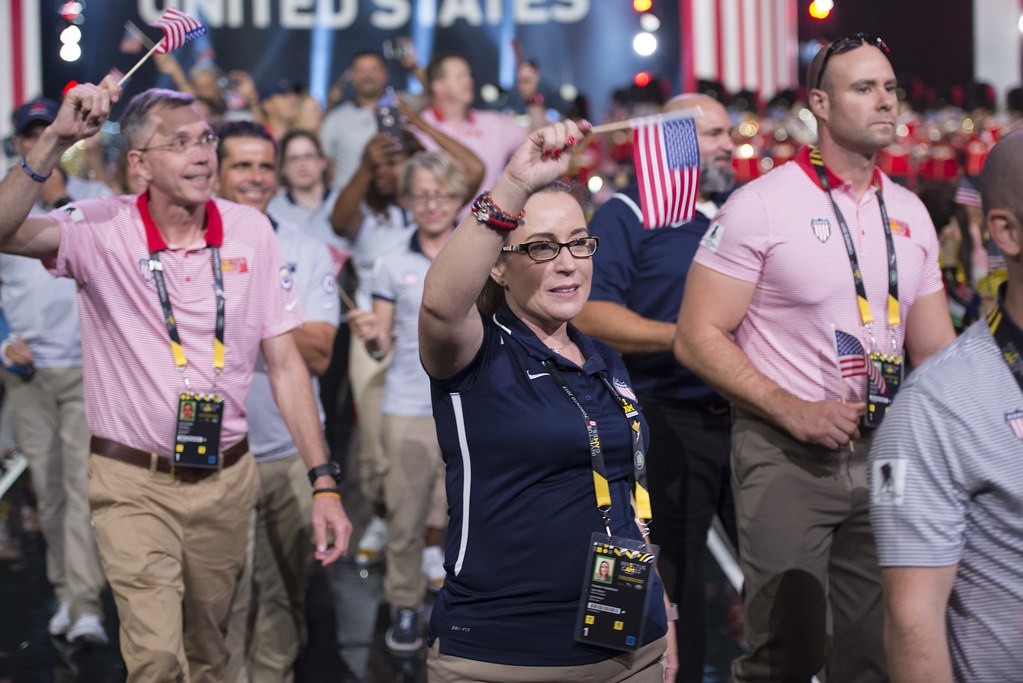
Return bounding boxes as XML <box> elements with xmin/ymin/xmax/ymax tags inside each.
<box><xmin>673</xmin><ymin>32</ymin><xmax>959</xmax><ymax>683</ymax></box>
<box><xmin>568</xmin><ymin>91</ymin><xmax>738</xmax><ymax>683</ymax></box>
<box><xmin>1</xmin><ymin>33</ymin><xmax>1023</xmax><ymax>683</ymax></box>
<box><xmin>866</xmin><ymin>128</ymin><xmax>1023</xmax><ymax>683</ymax></box>
<box><xmin>0</xmin><ymin>75</ymin><xmax>353</xmax><ymax>681</ymax></box>
<box><xmin>419</xmin><ymin>119</ymin><xmax>679</xmax><ymax>683</ymax></box>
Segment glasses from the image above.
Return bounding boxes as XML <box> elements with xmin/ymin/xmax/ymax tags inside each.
<box><xmin>139</xmin><ymin>133</ymin><xmax>222</xmax><ymax>156</ymax></box>
<box><xmin>815</xmin><ymin>30</ymin><xmax>892</xmax><ymax>89</ymax></box>
<box><xmin>408</xmin><ymin>186</ymin><xmax>459</xmax><ymax>204</ymax></box>
<box><xmin>501</xmin><ymin>236</ymin><xmax>599</xmax><ymax>264</ymax></box>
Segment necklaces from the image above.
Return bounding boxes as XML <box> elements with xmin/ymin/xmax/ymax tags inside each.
<box><xmin>548</xmin><ymin>339</ymin><xmax>571</xmax><ymax>354</ymax></box>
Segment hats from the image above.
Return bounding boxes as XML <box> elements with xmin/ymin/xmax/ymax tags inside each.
<box><xmin>15</xmin><ymin>98</ymin><xmax>57</xmax><ymax>134</ymax></box>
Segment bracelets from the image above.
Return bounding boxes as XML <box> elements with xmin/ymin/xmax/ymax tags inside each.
<box><xmin>19</xmin><ymin>154</ymin><xmax>53</xmax><ymax>182</ymax></box>
<box><xmin>312</xmin><ymin>492</ymin><xmax>341</xmax><ymax>498</ymax></box>
<box><xmin>471</xmin><ymin>191</ymin><xmax>526</xmax><ymax>231</ymax></box>
<box><xmin>312</xmin><ymin>488</ymin><xmax>344</xmax><ymax>494</ymax></box>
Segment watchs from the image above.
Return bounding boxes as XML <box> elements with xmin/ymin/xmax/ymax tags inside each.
<box><xmin>308</xmin><ymin>461</ymin><xmax>342</xmax><ymax>485</ymax></box>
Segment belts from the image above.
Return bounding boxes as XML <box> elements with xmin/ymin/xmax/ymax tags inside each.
<box><xmin>730</xmin><ymin>405</ymin><xmax>756</xmax><ymax>419</ymax></box>
<box><xmin>91</xmin><ymin>432</ymin><xmax>249</xmax><ymax>486</ymax></box>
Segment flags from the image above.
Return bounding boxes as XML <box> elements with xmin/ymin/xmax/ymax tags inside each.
<box><xmin>836</xmin><ymin>330</ymin><xmax>887</xmax><ymax>397</ymax></box>
<box><xmin>950</xmin><ymin>176</ymin><xmax>983</xmax><ymax>209</ymax></box>
<box><xmin>146</xmin><ymin>8</ymin><xmax>205</xmax><ymax>54</ymax></box>
<box><xmin>629</xmin><ymin>109</ymin><xmax>700</xmax><ymax>232</ymax></box>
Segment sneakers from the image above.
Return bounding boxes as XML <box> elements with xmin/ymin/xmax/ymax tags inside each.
<box><xmin>47</xmin><ymin>601</ymin><xmax>71</xmax><ymax>635</ymax></box>
<box><xmin>384</xmin><ymin>605</ymin><xmax>423</xmax><ymax>650</ymax></box>
<box><xmin>421</xmin><ymin>545</ymin><xmax>449</xmax><ymax>591</ymax></box>
<box><xmin>66</xmin><ymin>611</ymin><xmax>109</xmax><ymax>646</ymax></box>
<box><xmin>353</xmin><ymin>513</ymin><xmax>388</xmax><ymax>569</ymax></box>
